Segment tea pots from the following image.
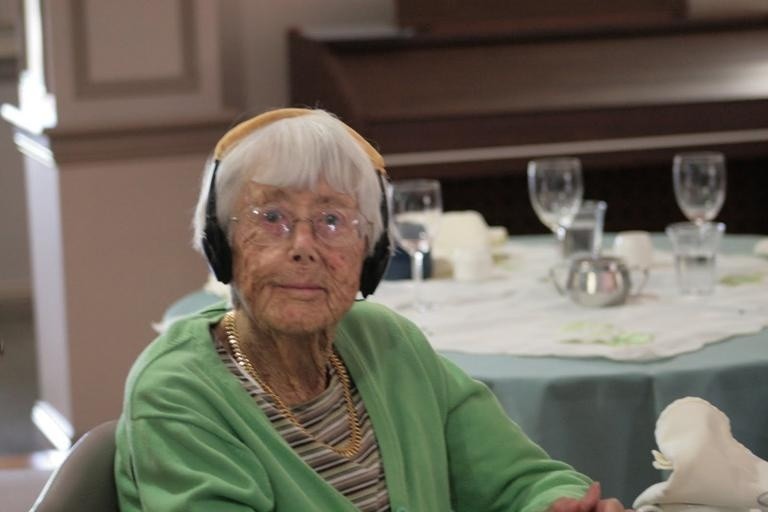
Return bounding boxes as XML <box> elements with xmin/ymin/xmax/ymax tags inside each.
<box><xmin>545</xmin><ymin>259</ymin><xmax>650</xmax><ymax>307</ymax></box>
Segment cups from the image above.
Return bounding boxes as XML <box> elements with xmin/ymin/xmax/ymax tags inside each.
<box><xmin>444</xmin><ymin>212</ymin><xmax>493</xmax><ymax>282</ymax></box>
<box><xmin>613</xmin><ymin>231</ymin><xmax>653</xmax><ymax>270</ymax></box>
<box><xmin>666</xmin><ymin>152</ymin><xmax>728</xmax><ymax>295</ymax></box>
<box><xmin>566</xmin><ymin>198</ymin><xmax>607</xmax><ymax>259</ymax></box>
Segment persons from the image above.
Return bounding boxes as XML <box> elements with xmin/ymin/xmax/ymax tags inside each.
<box><xmin>112</xmin><ymin>110</ymin><xmax>634</xmax><ymax>512</ymax></box>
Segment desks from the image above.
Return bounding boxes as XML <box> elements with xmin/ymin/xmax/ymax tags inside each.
<box><xmin>160</xmin><ymin>233</ymin><xmax>768</xmax><ymax>511</ymax></box>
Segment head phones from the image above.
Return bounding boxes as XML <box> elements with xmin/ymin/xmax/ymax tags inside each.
<box><xmin>204</xmin><ymin>108</ymin><xmax>391</xmax><ymax>298</ymax></box>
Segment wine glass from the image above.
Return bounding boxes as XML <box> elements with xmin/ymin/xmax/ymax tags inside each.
<box><xmin>386</xmin><ymin>180</ymin><xmax>442</xmax><ymax>314</ymax></box>
<box><xmin>528</xmin><ymin>157</ymin><xmax>584</xmax><ymax>254</ymax></box>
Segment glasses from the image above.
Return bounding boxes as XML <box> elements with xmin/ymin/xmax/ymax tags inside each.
<box><xmin>226</xmin><ymin>205</ymin><xmax>371</xmax><ymax>249</ymax></box>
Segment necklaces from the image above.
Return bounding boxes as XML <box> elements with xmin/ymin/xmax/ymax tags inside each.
<box><xmin>226</xmin><ymin>312</ymin><xmax>362</xmax><ymax>456</ymax></box>
